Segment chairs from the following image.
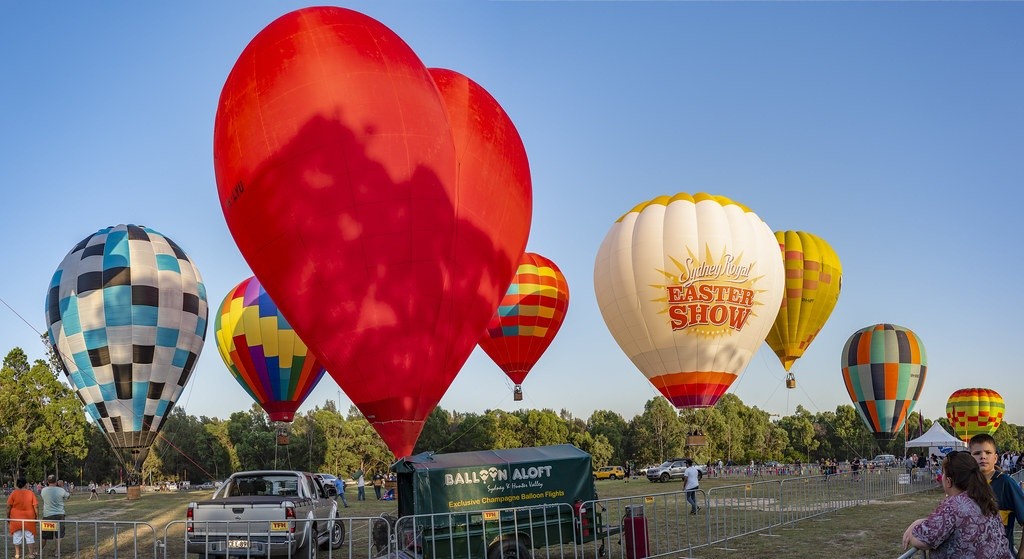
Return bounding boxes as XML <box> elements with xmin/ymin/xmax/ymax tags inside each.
<box><xmin>239</xmin><ymin>479</ymin><xmax>266</xmax><ymax>496</ymax></box>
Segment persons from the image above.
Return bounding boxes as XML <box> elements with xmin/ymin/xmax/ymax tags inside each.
<box><xmin>87</xmin><ymin>480</ymin><xmax>112</xmax><ymax>501</ymax></box>
<box><xmin>126</xmin><ymin>478</ymin><xmax>137</xmax><ymax>488</ymax></box>
<box><xmin>996</xmin><ymin>450</ymin><xmax>1024</xmax><ymax>476</ymax></box>
<box><xmin>686</xmin><ymin>429</ymin><xmax>703</xmax><ymax>436</ymax></box>
<box><xmin>334</xmin><ymin>470</ymin><xmax>397</xmax><ymax>508</ymax></box>
<box><xmin>593</xmin><ymin>476</ymin><xmax>606</xmax><ymax>512</ymax></box>
<box><xmin>7</xmin><ymin>478</ymin><xmax>39</xmax><ymax>559</ymax></box>
<box><xmin>3</xmin><ymin>480</ymin><xmax>74</xmax><ymax>495</ymax></box>
<box><xmin>706</xmin><ymin>457</ymin><xmax>803</xmax><ymax>478</ymax></box>
<box><xmin>894</xmin><ymin>453</ymin><xmax>942</xmax><ymax>487</ymax></box>
<box><xmin>682</xmin><ymin>459</ymin><xmax>701</xmax><ymax>514</ymax></box>
<box><xmin>623</xmin><ymin>461</ymin><xmax>631</xmax><ymax>483</ymax></box>
<box><xmin>903</xmin><ymin>451</ymin><xmax>1013</xmax><ymax>559</ymax></box>
<box><xmin>968</xmin><ymin>434</ymin><xmax>1024</xmax><ymax>559</ymax></box>
<box><xmin>33</xmin><ymin>475</ymin><xmax>70</xmax><ymax>557</ymax></box>
<box><xmin>816</xmin><ymin>457</ymin><xmax>891</xmax><ymax>482</ymax></box>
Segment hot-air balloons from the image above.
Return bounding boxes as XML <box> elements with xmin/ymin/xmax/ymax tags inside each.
<box><xmin>593</xmin><ymin>191</ymin><xmax>785</xmax><ymax>447</ymax></box>
<box><xmin>213</xmin><ymin>5</ymin><xmax>533</xmax><ymax>499</ymax></box>
<box><xmin>45</xmin><ymin>224</ymin><xmax>208</xmax><ymax>500</ymax></box>
<box><xmin>214</xmin><ymin>275</ymin><xmax>325</xmax><ymax>446</ymax></box>
<box><xmin>840</xmin><ymin>323</ymin><xmax>928</xmax><ymax>455</ymax></box>
<box><xmin>475</xmin><ymin>251</ymin><xmax>569</xmax><ymax>401</ymax></box>
<box><xmin>946</xmin><ymin>388</ymin><xmax>1006</xmax><ymax>442</ymax></box>
<box><xmin>765</xmin><ymin>230</ymin><xmax>843</xmax><ymax>388</ymax></box>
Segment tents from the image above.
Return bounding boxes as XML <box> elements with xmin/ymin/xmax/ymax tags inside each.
<box><xmin>905</xmin><ymin>421</ymin><xmax>967</xmax><ymax>479</ymax></box>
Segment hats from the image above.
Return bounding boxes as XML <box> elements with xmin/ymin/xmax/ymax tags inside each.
<box><xmin>684</xmin><ymin>459</ymin><xmax>693</xmax><ymax>464</ymax></box>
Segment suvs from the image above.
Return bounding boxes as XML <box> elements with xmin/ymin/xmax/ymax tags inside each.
<box><xmin>593</xmin><ymin>465</ymin><xmax>625</xmax><ymax>481</ymax></box>
<box><xmin>871</xmin><ymin>455</ymin><xmax>896</xmax><ymax>468</ymax></box>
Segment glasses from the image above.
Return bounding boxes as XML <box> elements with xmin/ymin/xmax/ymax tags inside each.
<box><xmin>947</xmin><ymin>450</ymin><xmax>971</xmax><ymax>476</ymax></box>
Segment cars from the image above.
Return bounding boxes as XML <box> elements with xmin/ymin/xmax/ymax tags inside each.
<box><xmin>281</xmin><ymin>474</ymin><xmax>347</xmax><ymax>494</ymax></box>
<box><xmin>155</xmin><ymin>483</ymin><xmax>178</xmax><ymax>491</ymax></box>
<box><xmin>106</xmin><ymin>483</ymin><xmax>128</xmax><ymax>495</ymax></box>
<box><xmin>766</xmin><ymin>461</ymin><xmax>780</xmax><ymax>467</ymax></box>
<box><xmin>195</xmin><ymin>483</ymin><xmax>215</xmax><ymax>491</ymax></box>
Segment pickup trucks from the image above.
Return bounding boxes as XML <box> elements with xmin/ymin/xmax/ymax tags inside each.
<box><xmin>637</xmin><ymin>465</ymin><xmax>658</xmax><ymax>475</ymax></box>
<box><xmin>646</xmin><ymin>459</ymin><xmax>708</xmax><ymax>483</ymax></box>
<box><xmin>184</xmin><ymin>470</ymin><xmax>345</xmax><ymax>559</ymax></box>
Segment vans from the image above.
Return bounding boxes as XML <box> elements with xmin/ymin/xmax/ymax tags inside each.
<box><xmin>178</xmin><ymin>481</ymin><xmax>191</xmax><ymax>489</ymax></box>
<box><xmin>213</xmin><ymin>481</ymin><xmax>223</xmax><ymax>489</ymax></box>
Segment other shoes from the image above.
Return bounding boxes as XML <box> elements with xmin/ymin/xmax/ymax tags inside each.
<box><xmin>690</xmin><ymin>511</ymin><xmax>696</xmax><ymax>514</ymax></box>
<box><xmin>696</xmin><ymin>506</ymin><xmax>701</xmax><ymax>512</ymax></box>
<box><xmin>345</xmin><ymin>505</ymin><xmax>350</xmax><ymax>508</ymax></box>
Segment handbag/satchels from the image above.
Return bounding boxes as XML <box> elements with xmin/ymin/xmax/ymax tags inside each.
<box><xmin>697</xmin><ymin>470</ymin><xmax>702</xmax><ymax>481</ymax></box>
